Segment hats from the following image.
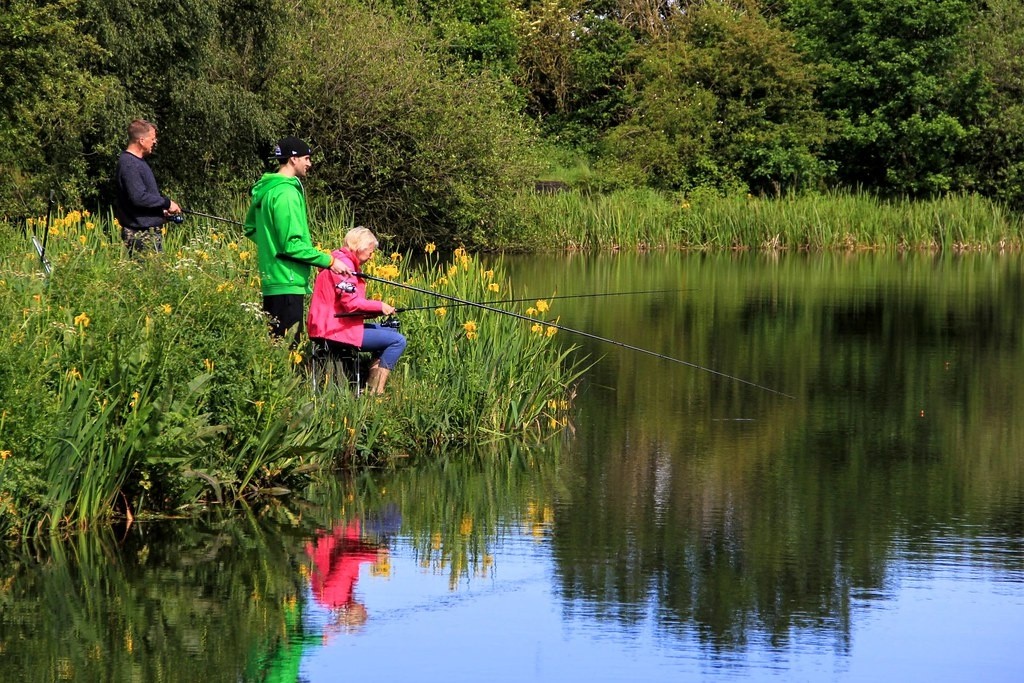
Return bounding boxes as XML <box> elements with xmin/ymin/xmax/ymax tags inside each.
<box><xmin>268</xmin><ymin>136</ymin><xmax>311</xmax><ymax>160</ymax></box>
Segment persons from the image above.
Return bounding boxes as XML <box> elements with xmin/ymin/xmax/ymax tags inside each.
<box><xmin>307</xmin><ymin>225</ymin><xmax>407</xmax><ymax>400</ymax></box>
<box><xmin>243</xmin><ymin>135</ymin><xmax>353</xmax><ymax>357</ymax></box>
<box><xmin>303</xmin><ymin>495</ymin><xmax>400</xmax><ymax>636</ymax></box>
<box><xmin>111</xmin><ymin>120</ymin><xmax>182</xmax><ymax>263</ymax></box>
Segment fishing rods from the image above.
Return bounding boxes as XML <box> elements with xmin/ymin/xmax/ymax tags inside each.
<box><xmin>163</xmin><ymin>208</ymin><xmax>245</xmax><ymax>226</ymax></box>
<box><xmin>333</xmin><ymin>289</ymin><xmax>705</xmax><ymax>318</ymax></box>
<box><xmin>275</xmin><ymin>250</ymin><xmax>799</xmax><ymax>400</ymax></box>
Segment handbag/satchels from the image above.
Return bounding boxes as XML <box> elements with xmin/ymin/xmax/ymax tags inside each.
<box><xmin>122</xmin><ymin>227</ymin><xmax>162</xmax><ymax>252</ymax></box>
